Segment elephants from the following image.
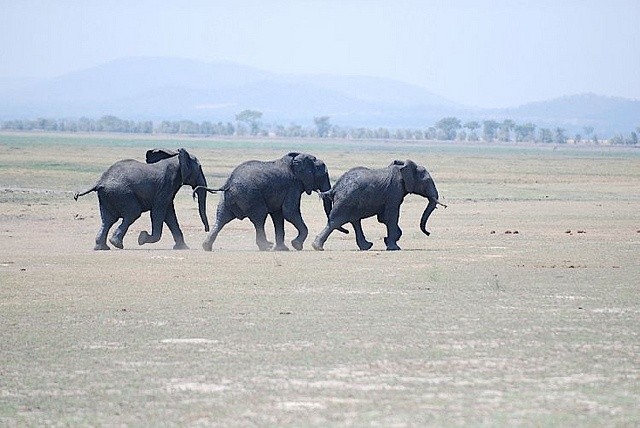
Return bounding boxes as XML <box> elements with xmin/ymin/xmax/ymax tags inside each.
<box><xmin>73</xmin><ymin>145</ymin><xmax>216</xmax><ymax>251</ymax></box>
<box><xmin>311</xmin><ymin>158</ymin><xmax>447</xmax><ymax>252</ymax></box>
<box><xmin>191</xmin><ymin>151</ymin><xmax>350</xmax><ymax>253</ymax></box>
